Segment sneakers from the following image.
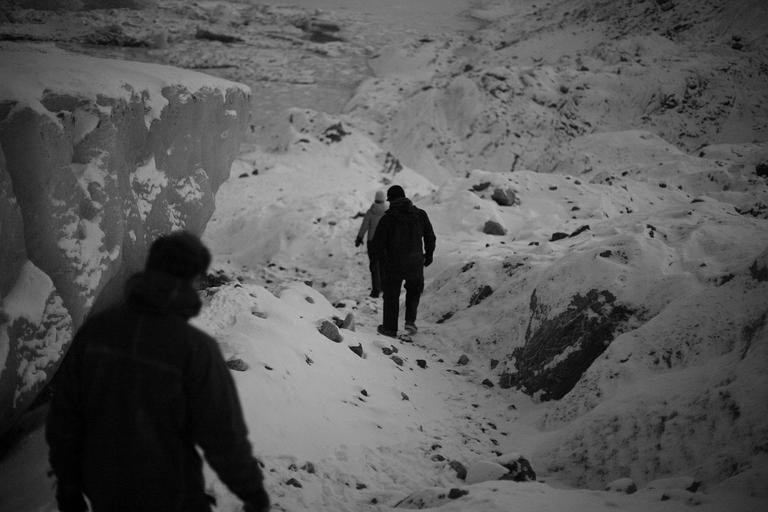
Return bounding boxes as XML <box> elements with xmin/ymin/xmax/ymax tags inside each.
<box><xmin>404</xmin><ymin>321</ymin><xmax>418</xmax><ymax>333</ymax></box>
<box><xmin>370</xmin><ymin>292</ymin><xmax>379</xmax><ymax>298</ymax></box>
<box><xmin>376</xmin><ymin>324</ymin><xmax>398</xmax><ymax>337</ymax></box>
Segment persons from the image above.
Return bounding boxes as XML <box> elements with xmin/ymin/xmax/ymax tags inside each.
<box><xmin>45</xmin><ymin>229</ymin><xmax>272</xmax><ymax>511</ymax></box>
<box><xmin>370</xmin><ymin>184</ymin><xmax>436</xmax><ymax>337</ymax></box>
<box><xmin>355</xmin><ymin>192</ymin><xmax>388</xmax><ymax>298</ymax></box>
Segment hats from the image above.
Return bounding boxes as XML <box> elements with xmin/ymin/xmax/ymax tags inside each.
<box><xmin>385</xmin><ymin>185</ymin><xmax>406</xmax><ymax>201</ymax></box>
<box><xmin>146</xmin><ymin>230</ymin><xmax>213</xmax><ymax>280</ymax></box>
<box><xmin>374</xmin><ymin>190</ymin><xmax>385</xmax><ymax>201</ymax></box>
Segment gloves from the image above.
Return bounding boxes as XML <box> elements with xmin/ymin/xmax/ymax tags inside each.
<box><xmin>355</xmin><ymin>236</ymin><xmax>364</xmax><ymax>247</ymax></box>
<box><xmin>241</xmin><ymin>497</ymin><xmax>270</xmax><ymax>512</ymax></box>
<box><xmin>423</xmin><ymin>251</ymin><xmax>434</xmax><ymax>267</ymax></box>
<box><xmin>370</xmin><ymin>260</ymin><xmax>379</xmax><ymax>275</ymax></box>
<box><xmin>53</xmin><ymin>477</ymin><xmax>89</xmax><ymax>512</ymax></box>
